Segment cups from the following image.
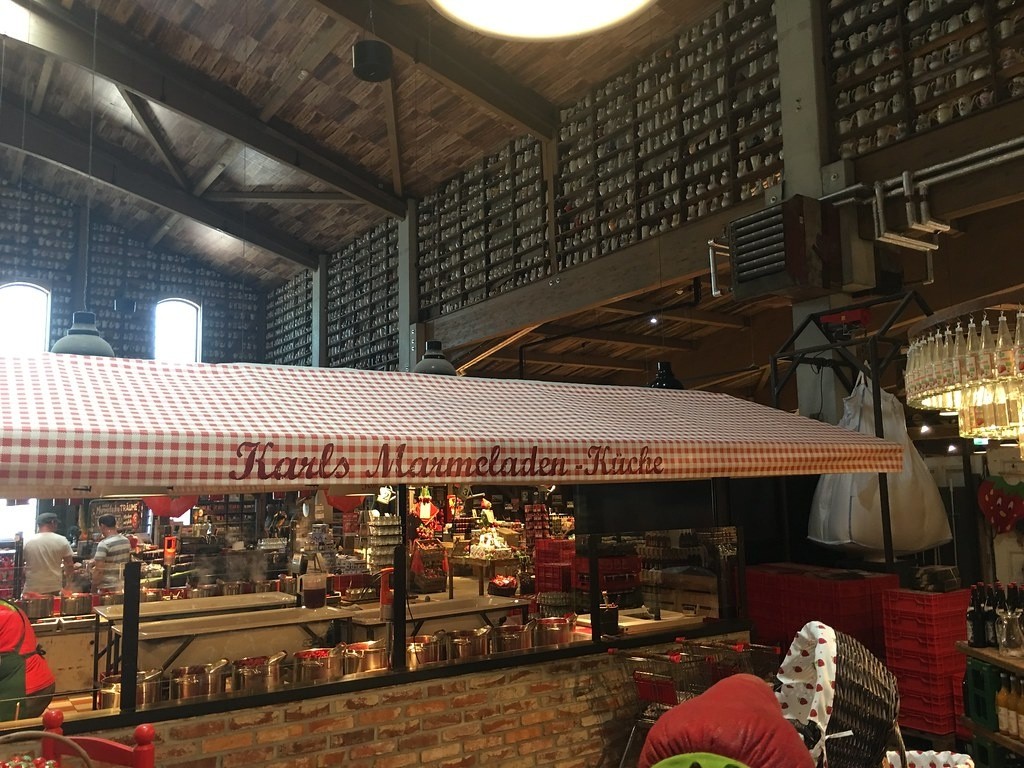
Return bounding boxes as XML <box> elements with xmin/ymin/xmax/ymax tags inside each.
<box><xmin>257</xmin><ymin>537</ymin><xmax>287</xmax><ymax>550</ymax></box>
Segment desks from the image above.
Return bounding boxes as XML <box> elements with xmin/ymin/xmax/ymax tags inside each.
<box><xmin>340</xmin><ymin>594</ymin><xmax>532</xmax><ymax>641</ymax></box>
<box><xmin>110</xmin><ymin>605</ymin><xmax>356</xmax><ymax>675</ymax></box>
<box><xmin>448</xmin><ymin>556</ymin><xmax>523</xmax><ymax>599</ymax></box>
<box><xmin>92</xmin><ymin>591</ymin><xmax>297</xmax><ymax>711</ymax></box>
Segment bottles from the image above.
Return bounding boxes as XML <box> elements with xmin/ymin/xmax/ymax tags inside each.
<box><xmin>303</xmin><ymin>525</ymin><xmax>334</xmax><ymax>550</ymax></box>
<box><xmin>193</xmin><ymin>519</ymin><xmax>213</xmax><ymax>536</ymax></box>
<box><xmin>536</xmin><ymin>591</ymin><xmax>571</xmax><ymax>618</ymax></box>
<box><xmin>966</xmin><ymin>580</ymin><xmax>1024</xmax><ymax>648</ymax></box>
<box><xmin>904</xmin><ymin>314</ymin><xmax>1024</xmax><ymax>461</ymax></box>
<box><xmin>638</xmin><ymin>531</ymin><xmax>736</xmax><ymax>570</ymax></box>
<box><xmin>997</xmin><ymin>673</ymin><xmax>1024</xmax><ymax>743</ymax></box>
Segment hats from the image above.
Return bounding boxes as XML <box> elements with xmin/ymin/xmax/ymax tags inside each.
<box><xmin>37</xmin><ymin>513</ymin><xmax>61</xmax><ymax>524</ymax></box>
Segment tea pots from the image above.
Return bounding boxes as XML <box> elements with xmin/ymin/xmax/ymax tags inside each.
<box><xmin>0</xmin><ymin>1</ymin><xmax>1024</xmax><ymax>371</ymax></box>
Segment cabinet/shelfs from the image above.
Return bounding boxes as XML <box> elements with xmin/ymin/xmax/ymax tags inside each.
<box><xmin>555</xmin><ymin>0</ymin><xmax>784</xmax><ymax>273</ymax></box>
<box><xmin>190</xmin><ymin>493</ymin><xmax>269</xmax><ymax>543</ymax></box>
<box><xmin>818</xmin><ymin>0</ymin><xmax>1024</xmax><ymax>164</ymax></box>
<box><xmin>265</xmin><ymin>268</ymin><xmax>313</xmax><ymax>366</ymax></box>
<box><xmin>415</xmin><ymin>139</ymin><xmax>552</xmax><ymax>323</ymax></box>
<box><xmin>87</xmin><ymin>215</ymin><xmax>261</xmax><ymax>363</ymax></box>
<box><xmin>367</xmin><ymin>524</ymin><xmax>403</xmax><ymax>568</ymax></box>
<box><xmin>325</xmin><ymin>217</ymin><xmax>399</xmax><ymax>371</ymax></box>
<box><xmin>953</xmin><ymin>640</ymin><xmax>1024</xmax><ymax>756</ymax></box>
<box><xmin>0</xmin><ymin>175</ymin><xmax>81</xmax><ymax>354</ymax></box>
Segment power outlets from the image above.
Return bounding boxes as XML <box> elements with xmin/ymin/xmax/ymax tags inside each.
<box><xmin>809</xmin><ymin>412</ymin><xmax>824</xmax><ymax>422</ymax></box>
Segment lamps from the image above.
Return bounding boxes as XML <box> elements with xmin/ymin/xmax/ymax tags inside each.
<box><xmin>413</xmin><ymin>6</ymin><xmax>457</xmax><ymax>377</ymax></box>
<box><xmin>957</xmin><ymin>399</ymin><xmax>1024</xmax><ymax>440</ymax></box>
<box><xmin>645</xmin><ymin>8</ymin><xmax>685</xmax><ymax>390</ymax></box>
<box><xmin>50</xmin><ymin>0</ymin><xmax>117</xmax><ymax>358</ymax></box>
<box><xmin>903</xmin><ymin>223</ymin><xmax>1024</xmax><ymax>413</ymax></box>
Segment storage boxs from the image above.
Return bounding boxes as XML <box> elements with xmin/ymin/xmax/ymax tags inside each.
<box><xmin>972</xmin><ymin>731</ymin><xmax>1024</xmax><ymax>768</ymax></box>
<box><xmin>965</xmin><ymin>656</ymin><xmax>1015</xmax><ymax>731</ymax></box>
<box><xmin>534</xmin><ymin>538</ymin><xmax>642</xmax><ymax>594</ymax></box>
<box><xmin>507</xmin><ymin>594</ymin><xmax>540</xmax><ymax>617</ymax></box>
<box><xmin>744</xmin><ymin>561</ymin><xmax>974</xmax><ymax>739</ymax></box>
<box><xmin>498</xmin><ymin>528</ymin><xmax>520</xmax><ymax>548</ymax></box>
<box><xmin>415</xmin><ymin>538</ymin><xmax>445</xmax><ymax>563</ymax></box>
<box><xmin>414</xmin><ymin>568</ymin><xmax>448</xmax><ymax>593</ymax></box>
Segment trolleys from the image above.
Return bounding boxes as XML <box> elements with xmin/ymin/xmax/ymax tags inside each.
<box><xmin>606</xmin><ymin>636</ymin><xmax>781</xmax><ymax>768</ymax></box>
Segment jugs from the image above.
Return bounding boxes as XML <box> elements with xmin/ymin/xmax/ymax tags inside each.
<box><xmin>995</xmin><ymin>611</ymin><xmax>1024</xmax><ymax>658</ymax></box>
<box><xmin>297</xmin><ymin>572</ymin><xmax>328</xmax><ymax>609</ymax></box>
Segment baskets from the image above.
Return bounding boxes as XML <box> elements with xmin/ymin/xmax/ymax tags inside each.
<box><xmin>773</xmin><ymin>621</ymin><xmax>908</xmax><ymax>768</ymax></box>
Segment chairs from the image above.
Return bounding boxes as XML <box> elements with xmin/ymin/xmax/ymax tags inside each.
<box><xmin>42</xmin><ymin>709</ymin><xmax>156</xmax><ymax>768</ymax></box>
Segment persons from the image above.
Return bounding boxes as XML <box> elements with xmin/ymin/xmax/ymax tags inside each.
<box><xmin>20</xmin><ymin>513</ymin><xmax>75</xmax><ymax>598</ymax></box>
<box><xmin>0</xmin><ymin>600</ymin><xmax>55</xmax><ymax>721</ymax></box>
<box><xmin>264</xmin><ymin>504</ymin><xmax>287</xmax><ymax>538</ymax></box>
<box><xmin>501</xmin><ymin>494</ymin><xmax>526</xmax><ymax>523</ymax></box>
<box><xmin>90</xmin><ymin>514</ymin><xmax>132</xmax><ymax>594</ymax></box>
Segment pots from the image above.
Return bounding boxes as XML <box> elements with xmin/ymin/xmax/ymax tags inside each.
<box><xmin>20</xmin><ymin>577</ymin><xmax>296</xmax><ymax>618</ymax></box>
<box><xmin>101</xmin><ymin>619</ymin><xmax>574</xmax><ymax>710</ymax></box>
